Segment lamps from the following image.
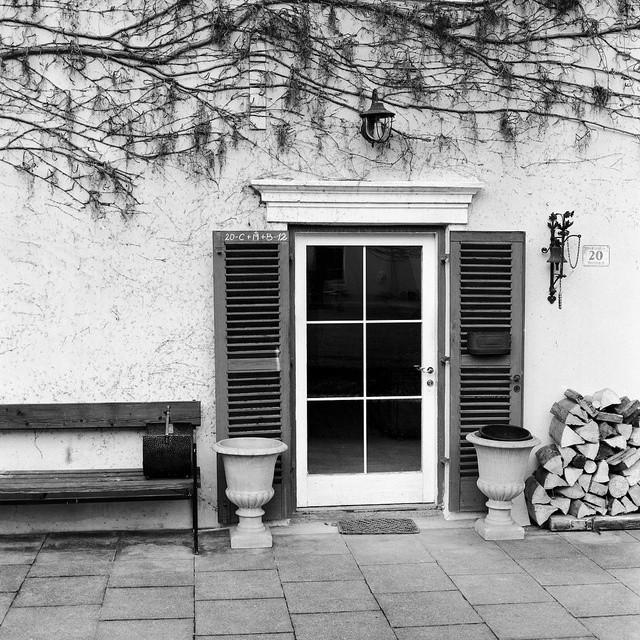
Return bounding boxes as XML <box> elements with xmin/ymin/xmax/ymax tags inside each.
<box><xmin>541</xmin><ymin>210</ymin><xmax>581</xmax><ymax>311</ymax></box>
<box><xmin>357</xmin><ymin>88</ymin><xmax>396</xmax><ymax>148</ymax></box>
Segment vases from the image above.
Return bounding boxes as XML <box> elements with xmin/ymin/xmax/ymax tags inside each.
<box><xmin>465</xmin><ymin>427</ymin><xmax>542</xmax><ymax>542</ymax></box>
<box><xmin>211</xmin><ymin>436</ymin><xmax>289</xmax><ymax>549</ymax></box>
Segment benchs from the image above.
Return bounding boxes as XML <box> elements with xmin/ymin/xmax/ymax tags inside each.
<box><xmin>0</xmin><ymin>400</ymin><xmax>202</xmax><ymax>555</ymax></box>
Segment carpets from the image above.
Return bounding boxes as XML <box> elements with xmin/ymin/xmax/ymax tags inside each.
<box><xmin>336</xmin><ymin>517</ymin><xmax>421</xmax><ymax>535</ymax></box>
